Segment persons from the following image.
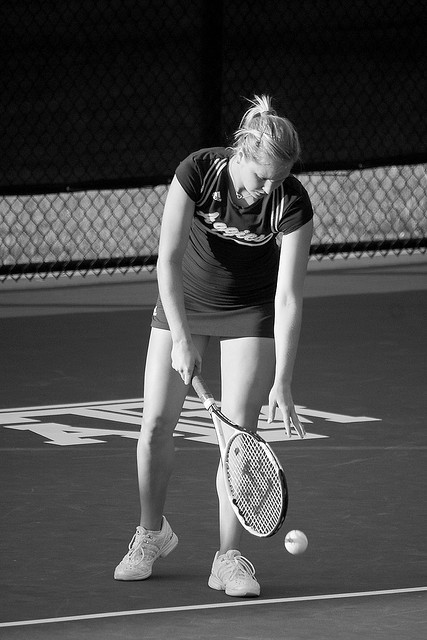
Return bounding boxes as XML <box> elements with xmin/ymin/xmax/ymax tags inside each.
<box><xmin>112</xmin><ymin>92</ymin><xmax>315</xmax><ymax>599</ymax></box>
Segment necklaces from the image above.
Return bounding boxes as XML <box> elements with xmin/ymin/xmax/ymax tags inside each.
<box><xmin>233</xmin><ymin>192</ymin><xmax>252</xmax><ymax>200</ymax></box>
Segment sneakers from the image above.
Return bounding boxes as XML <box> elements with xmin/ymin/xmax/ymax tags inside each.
<box><xmin>209</xmin><ymin>549</ymin><xmax>261</xmax><ymax>596</ymax></box>
<box><xmin>115</xmin><ymin>515</ymin><xmax>178</xmax><ymax>579</ymax></box>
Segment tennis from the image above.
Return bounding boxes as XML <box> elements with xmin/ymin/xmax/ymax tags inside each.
<box><xmin>283</xmin><ymin>529</ymin><xmax>309</xmax><ymax>556</ymax></box>
